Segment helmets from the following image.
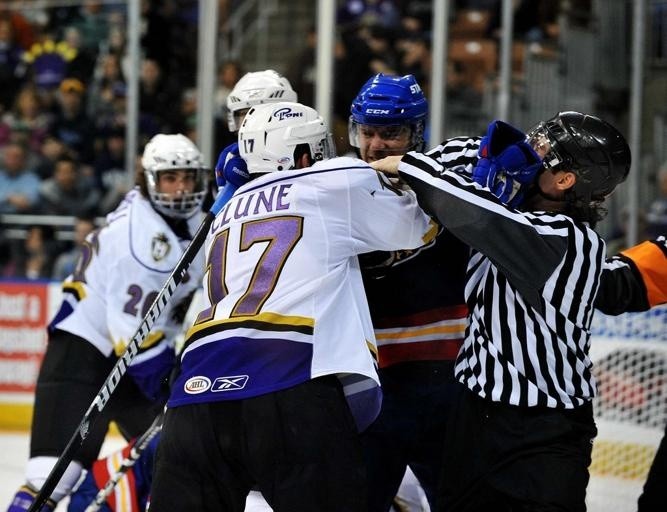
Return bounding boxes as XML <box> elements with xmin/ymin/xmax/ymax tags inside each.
<box><xmin>352</xmin><ymin>73</ymin><xmax>427</xmax><ymax>148</ymax></box>
<box><xmin>226</xmin><ymin>69</ymin><xmax>327</xmax><ymax>173</ymax></box>
<box><xmin>539</xmin><ymin>111</ymin><xmax>632</xmax><ymax>212</ymax></box>
<box><xmin>141</xmin><ymin>133</ymin><xmax>202</xmax><ymax>192</ymax></box>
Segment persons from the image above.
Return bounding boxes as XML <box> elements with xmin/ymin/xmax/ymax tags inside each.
<box><xmin>226</xmin><ymin>69</ymin><xmax>297</xmax><ymax>132</ymax></box>
<box><xmin>214</xmin><ymin>73</ymin><xmax>486</xmax><ymax>512</ymax></box>
<box><xmin>291</xmin><ymin>0</ymin><xmax>667</xmax><ymax>235</ymax></box>
<box><xmin>147</xmin><ymin>103</ymin><xmax>543</xmax><ymax>512</ymax></box>
<box><xmin>595</xmin><ymin>234</ymin><xmax>667</xmax><ymax>512</ymax></box>
<box><xmin>370</xmin><ymin>111</ymin><xmax>631</xmax><ymax>512</ymax></box>
<box><xmin>8</xmin><ymin>134</ymin><xmax>207</xmax><ymax>512</ymax></box>
<box><xmin>0</xmin><ymin>1</ymin><xmax>246</xmax><ymax>278</ymax></box>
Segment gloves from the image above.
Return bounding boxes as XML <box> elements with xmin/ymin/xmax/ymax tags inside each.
<box><xmin>473</xmin><ymin>120</ymin><xmax>541</xmax><ymax>207</ymax></box>
<box><xmin>215</xmin><ymin>142</ymin><xmax>251</xmax><ymax>187</ymax></box>
